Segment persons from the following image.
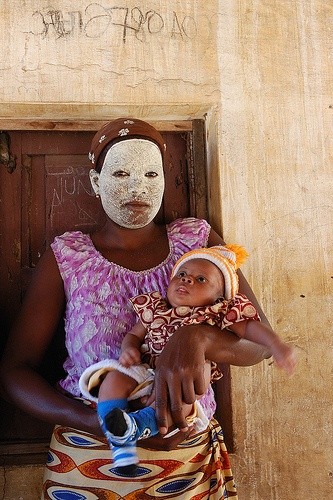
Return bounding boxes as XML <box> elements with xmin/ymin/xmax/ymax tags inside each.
<box><xmin>77</xmin><ymin>242</ymin><xmax>297</xmax><ymax>476</ymax></box>
<box><xmin>1</xmin><ymin>116</ymin><xmax>273</xmax><ymax>500</ymax></box>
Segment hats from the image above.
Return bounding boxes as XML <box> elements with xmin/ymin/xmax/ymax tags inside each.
<box><xmin>170</xmin><ymin>243</ymin><xmax>250</xmax><ymax>300</ymax></box>
<box><xmin>88</xmin><ymin>117</ymin><xmax>166</xmax><ymax>173</ymax></box>
